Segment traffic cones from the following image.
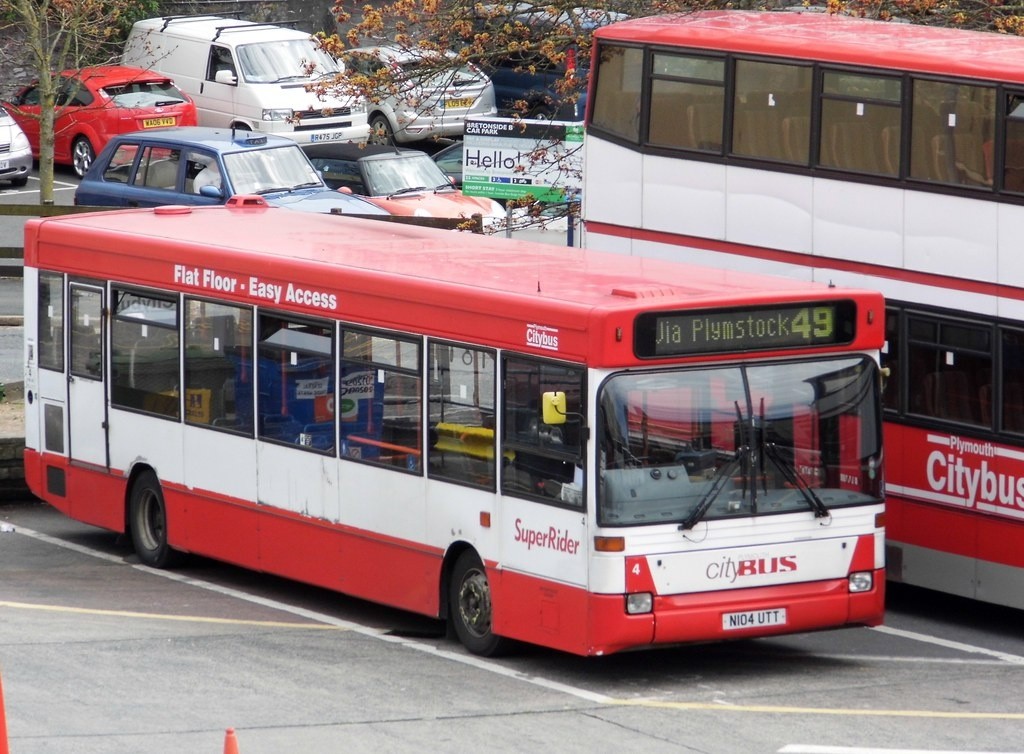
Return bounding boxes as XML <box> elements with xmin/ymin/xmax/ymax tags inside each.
<box><xmin>224</xmin><ymin>729</ymin><xmax>238</xmax><ymax>754</ymax></box>
<box><xmin>0</xmin><ymin>674</ymin><xmax>9</xmax><ymax>753</ymax></box>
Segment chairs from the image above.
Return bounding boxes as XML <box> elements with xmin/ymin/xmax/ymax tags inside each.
<box><xmin>686</xmin><ymin>100</ymin><xmax>986</xmax><ymax>185</ymax></box>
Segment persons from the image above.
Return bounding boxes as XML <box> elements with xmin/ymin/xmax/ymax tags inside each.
<box><xmin>192</xmin><ymin>158</ymin><xmax>221</xmax><ymax>196</ymax></box>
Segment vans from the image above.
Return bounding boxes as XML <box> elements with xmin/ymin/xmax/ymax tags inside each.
<box><xmin>121</xmin><ymin>12</ymin><xmax>372</xmax><ymax>149</ymax></box>
<box><xmin>427</xmin><ymin>8</ymin><xmax>631</xmax><ymax>122</ymax></box>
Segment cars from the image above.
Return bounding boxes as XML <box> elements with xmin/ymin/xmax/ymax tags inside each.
<box><xmin>0</xmin><ymin>108</ymin><xmax>34</xmax><ymax>187</ymax></box>
<box><xmin>74</xmin><ymin>125</ymin><xmax>392</xmax><ymax>217</ymax></box>
<box><xmin>333</xmin><ymin>42</ymin><xmax>498</xmax><ymax>146</ymax></box>
<box><xmin>2</xmin><ymin>66</ymin><xmax>197</xmax><ymax>181</ymax></box>
<box><xmin>301</xmin><ymin>142</ymin><xmax>506</xmax><ymax>235</ymax></box>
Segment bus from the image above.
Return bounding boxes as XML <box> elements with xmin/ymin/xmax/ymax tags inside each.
<box><xmin>24</xmin><ymin>196</ymin><xmax>889</xmax><ymax>657</ymax></box>
<box><xmin>582</xmin><ymin>9</ymin><xmax>1024</xmax><ymax>613</ymax></box>
